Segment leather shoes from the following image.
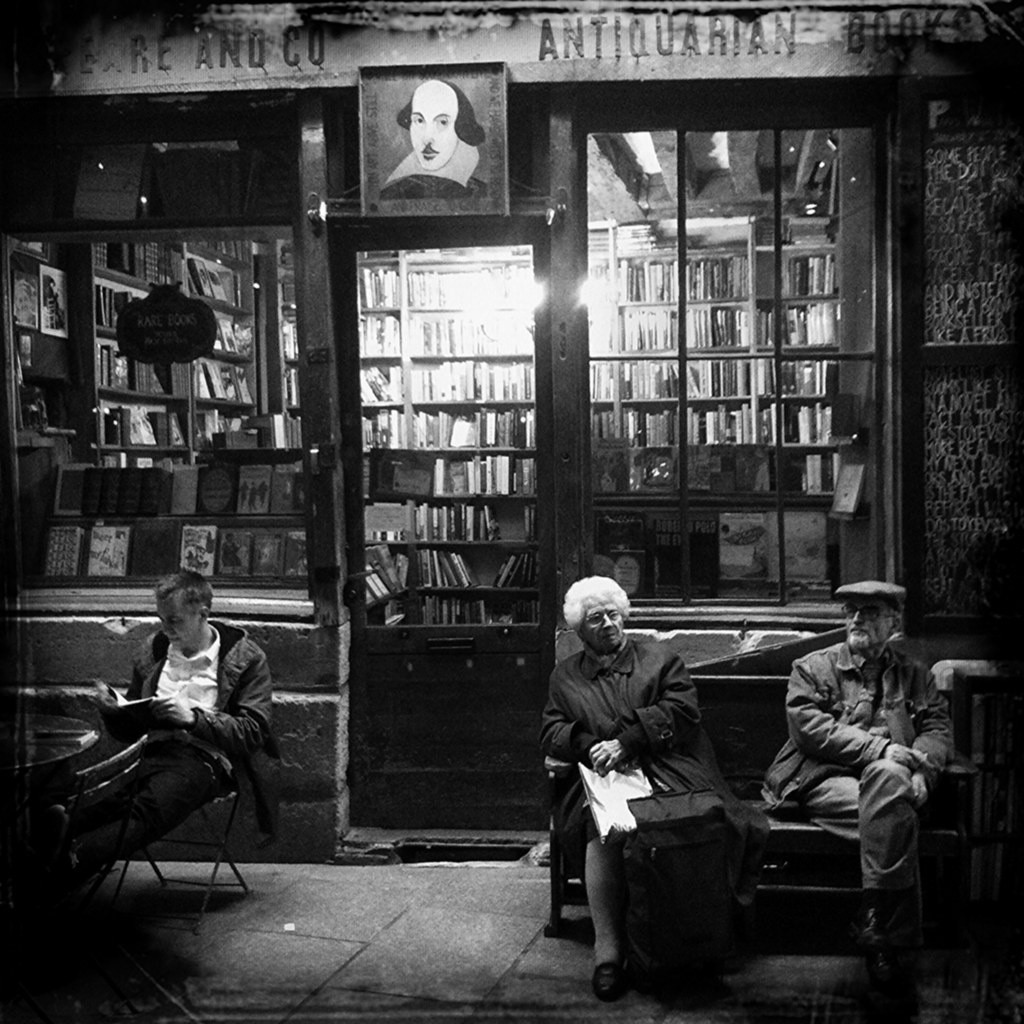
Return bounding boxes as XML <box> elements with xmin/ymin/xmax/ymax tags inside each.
<box><xmin>592</xmin><ymin>944</ymin><xmax>627</xmax><ymax>1001</ymax></box>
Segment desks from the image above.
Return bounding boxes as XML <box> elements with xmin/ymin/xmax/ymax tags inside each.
<box><xmin>0</xmin><ymin>715</ymin><xmax>100</xmax><ymax>950</ymax></box>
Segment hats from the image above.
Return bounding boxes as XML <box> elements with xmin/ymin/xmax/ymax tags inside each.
<box><xmin>834</xmin><ymin>580</ymin><xmax>906</xmax><ymax>610</ymax></box>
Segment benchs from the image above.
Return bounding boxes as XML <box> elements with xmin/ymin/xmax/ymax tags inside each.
<box><xmin>544</xmin><ymin>674</ymin><xmax>964</xmax><ymax>940</ymax></box>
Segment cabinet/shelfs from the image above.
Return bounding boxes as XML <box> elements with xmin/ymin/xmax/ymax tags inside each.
<box><xmin>11</xmin><ymin>215</ymin><xmax>841</xmax><ymax>628</ymax></box>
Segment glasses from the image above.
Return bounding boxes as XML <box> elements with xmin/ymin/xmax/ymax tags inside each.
<box><xmin>585</xmin><ymin>612</ymin><xmax>620</xmax><ymax>624</ymax></box>
<box><xmin>841</xmin><ymin>603</ymin><xmax>896</xmax><ymax>621</ymax></box>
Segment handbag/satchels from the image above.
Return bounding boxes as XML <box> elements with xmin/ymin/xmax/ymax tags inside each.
<box><xmin>577</xmin><ymin>762</ymin><xmax>733</xmax><ymax>851</ymax></box>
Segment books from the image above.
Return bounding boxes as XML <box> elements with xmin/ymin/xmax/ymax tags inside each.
<box><xmin>0</xmin><ymin>230</ymin><xmax>840</xmax><ymax>628</ymax></box>
<box><xmin>88</xmin><ymin>675</ymin><xmax>156</xmax><ymax>734</ymax></box>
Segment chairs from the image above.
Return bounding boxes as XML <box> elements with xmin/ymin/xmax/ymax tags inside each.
<box><xmin>0</xmin><ymin>733</ymin><xmax>202</xmax><ymax>1024</ymax></box>
<box><xmin>99</xmin><ymin>743</ymin><xmax>256</xmax><ymax>936</ymax></box>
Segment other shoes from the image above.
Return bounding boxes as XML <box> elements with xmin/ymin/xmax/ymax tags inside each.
<box><xmin>866</xmin><ymin>948</ymin><xmax>903</xmax><ymax>990</ymax></box>
<box><xmin>856</xmin><ymin>908</ymin><xmax>890</xmax><ymax>948</ymax></box>
<box><xmin>38</xmin><ymin>804</ymin><xmax>70</xmax><ymax>857</ymax></box>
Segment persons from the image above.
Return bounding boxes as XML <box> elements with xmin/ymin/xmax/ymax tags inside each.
<box><xmin>760</xmin><ymin>581</ymin><xmax>955</xmax><ymax>986</ymax></box>
<box><xmin>33</xmin><ymin>570</ymin><xmax>271</xmax><ymax>875</ymax></box>
<box><xmin>538</xmin><ymin>574</ymin><xmax>770</xmax><ymax>1001</ymax></box>
